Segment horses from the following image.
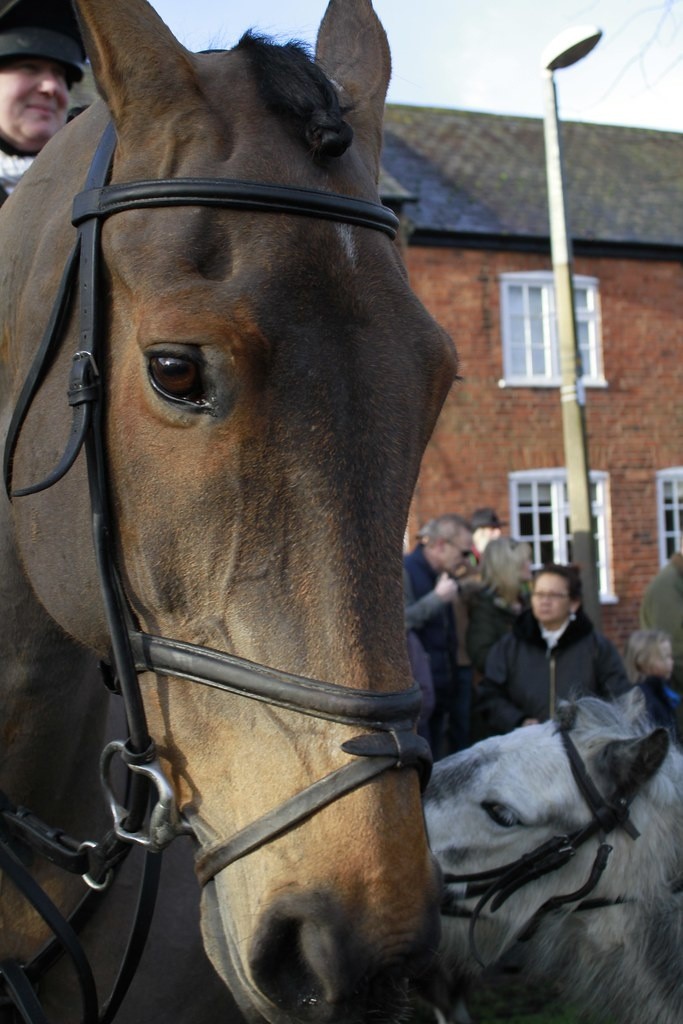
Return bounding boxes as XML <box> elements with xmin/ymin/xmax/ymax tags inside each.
<box><xmin>0</xmin><ymin>0</ymin><xmax>683</xmax><ymax>1024</ymax></box>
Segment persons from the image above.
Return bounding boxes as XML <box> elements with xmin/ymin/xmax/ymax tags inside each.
<box><xmin>620</xmin><ymin>628</ymin><xmax>681</xmax><ymax>750</ymax></box>
<box><xmin>483</xmin><ymin>565</ymin><xmax>632</xmax><ymax>734</ymax></box>
<box><xmin>401</xmin><ymin>508</ymin><xmax>534</xmax><ymax>776</ymax></box>
<box><xmin>0</xmin><ymin>0</ymin><xmax>95</xmax><ymax>215</ymax></box>
<box><xmin>639</xmin><ymin>519</ymin><xmax>683</xmax><ymax>691</ymax></box>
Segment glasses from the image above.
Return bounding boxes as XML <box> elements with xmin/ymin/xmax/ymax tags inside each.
<box><xmin>532</xmin><ymin>591</ymin><xmax>571</xmax><ymax>600</ymax></box>
<box><xmin>442</xmin><ymin>534</ymin><xmax>471</xmax><ymax>558</ymax></box>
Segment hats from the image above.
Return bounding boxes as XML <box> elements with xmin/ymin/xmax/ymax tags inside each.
<box><xmin>466</xmin><ymin>507</ymin><xmax>510</xmax><ymax>530</ymax></box>
<box><xmin>0</xmin><ymin>0</ymin><xmax>88</xmax><ymax>83</ymax></box>
